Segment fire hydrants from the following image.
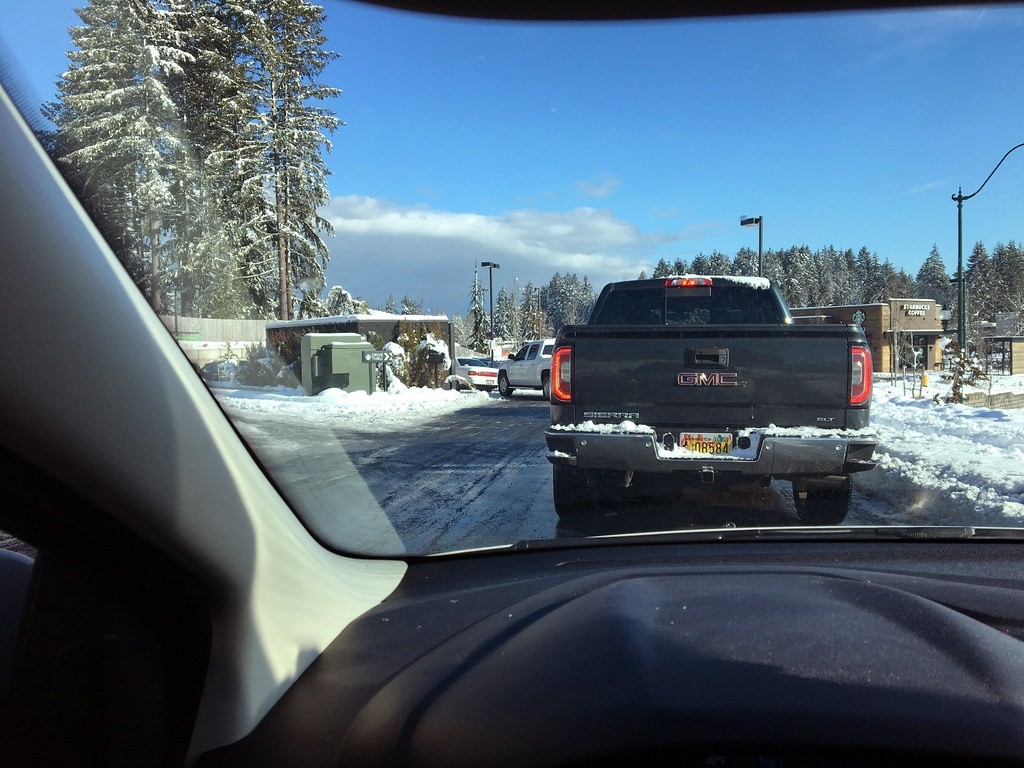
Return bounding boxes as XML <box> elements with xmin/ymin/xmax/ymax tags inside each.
<box><xmin>920</xmin><ymin>371</ymin><xmax>929</xmax><ymax>389</ymax></box>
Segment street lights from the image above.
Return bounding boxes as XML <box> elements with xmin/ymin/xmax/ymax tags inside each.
<box><xmin>741</xmin><ymin>215</ymin><xmax>763</xmax><ymax>277</ymax></box>
<box><xmin>481</xmin><ymin>261</ymin><xmax>501</xmax><ymax>368</ymax></box>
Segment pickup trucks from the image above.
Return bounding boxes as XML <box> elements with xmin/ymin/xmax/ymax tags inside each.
<box><xmin>498</xmin><ymin>337</ymin><xmax>556</xmax><ymax>401</ymax></box>
<box><xmin>543</xmin><ymin>274</ymin><xmax>877</xmax><ymax>526</ymax></box>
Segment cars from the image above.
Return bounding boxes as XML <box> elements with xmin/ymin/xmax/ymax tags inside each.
<box><xmin>455</xmin><ymin>356</ymin><xmax>499</xmax><ymax>392</ymax></box>
